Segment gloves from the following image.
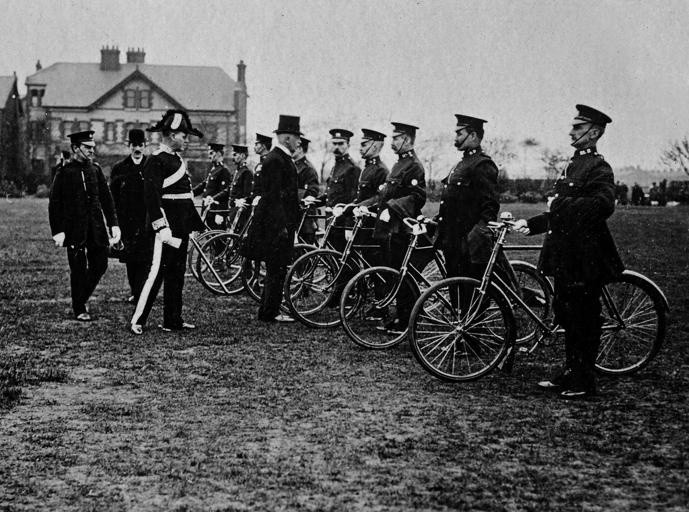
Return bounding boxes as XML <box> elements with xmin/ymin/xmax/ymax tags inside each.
<box><xmin>159</xmin><ymin>228</ymin><xmax>171</xmax><ymax>243</ymax></box>
<box><xmin>52</xmin><ymin>232</ymin><xmax>66</xmax><ymax>246</ymax></box>
<box><xmin>109</xmin><ymin>226</ymin><xmax>121</xmax><ymax>244</ymax></box>
<box><xmin>512</xmin><ymin>219</ymin><xmax>531</xmax><ymax>235</ymax></box>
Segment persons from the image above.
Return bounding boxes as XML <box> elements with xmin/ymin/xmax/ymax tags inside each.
<box><xmin>235</xmin><ymin>133</ymin><xmax>273</xmax><ymax>208</ymax></box>
<box><xmin>189</xmin><ymin>139</ymin><xmax>232</xmax><ymax>269</ymax></box>
<box><xmin>289</xmin><ymin>132</ymin><xmax>319</xmax><ymax>298</ymax></box>
<box><xmin>512</xmin><ymin>103</ymin><xmax>626</xmax><ymax>399</ymax></box>
<box><xmin>237</xmin><ymin>111</ymin><xmax>317</xmax><ymax>325</ymax></box>
<box><xmin>202</xmin><ymin>141</ymin><xmax>253</xmax><ymax>253</ymax></box>
<box><xmin>46</xmin><ymin>129</ymin><xmax>123</xmax><ymax>322</ymax></box>
<box><xmin>300</xmin><ymin>128</ymin><xmax>361</xmax><ymax>308</ymax></box>
<box><xmin>412</xmin><ymin>111</ymin><xmax>500</xmax><ymax>360</ymax></box>
<box><xmin>48</xmin><ymin>148</ymin><xmax>72</xmax><ymax>247</ymax></box>
<box><xmin>352</xmin><ymin>111</ymin><xmax>427</xmax><ymax>335</ymax></box>
<box><xmin>331</xmin><ymin>128</ymin><xmax>392</xmax><ymax>321</ymax></box>
<box><xmin>108</xmin><ymin>128</ymin><xmax>159</xmax><ymax>305</ymax></box>
<box><xmin>125</xmin><ymin>106</ymin><xmax>208</xmax><ymax>337</ymax></box>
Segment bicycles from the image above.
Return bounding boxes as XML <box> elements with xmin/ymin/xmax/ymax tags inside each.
<box><xmin>284</xmin><ymin>206</ymin><xmax>487</xmax><ymax>333</ymax></box>
<box><xmin>339</xmin><ymin>211</ymin><xmax>553</xmax><ymax>352</ymax></box>
<box><xmin>407</xmin><ymin>213</ymin><xmax>670</xmax><ymax>387</ymax></box>
<box><xmin>175</xmin><ymin>190</ymin><xmax>379</xmax><ymax>319</ymax></box>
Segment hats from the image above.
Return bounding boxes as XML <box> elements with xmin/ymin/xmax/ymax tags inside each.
<box><xmin>454</xmin><ymin>114</ymin><xmax>487</xmax><ymax>131</ymax></box>
<box><xmin>125</xmin><ymin>129</ymin><xmax>148</xmax><ymax>143</ymax></box>
<box><xmin>572</xmin><ymin>104</ymin><xmax>612</xmax><ymax>125</ymax></box>
<box><xmin>67</xmin><ymin>131</ymin><xmax>95</xmax><ymax>145</ymax></box>
<box><xmin>256</xmin><ymin>114</ymin><xmax>310</xmax><ymax>153</ymax></box>
<box><xmin>148</xmin><ymin>109</ymin><xmax>203</xmax><ymax>138</ymax></box>
<box><xmin>390</xmin><ymin>122</ymin><xmax>419</xmax><ymax>137</ymax></box>
<box><xmin>209</xmin><ymin>144</ymin><xmax>225</xmax><ymax>150</ymax></box>
<box><xmin>232</xmin><ymin>145</ymin><xmax>248</xmax><ymax>152</ymax></box>
<box><xmin>360</xmin><ymin>129</ymin><xmax>386</xmax><ymax>144</ymax></box>
<box><xmin>329</xmin><ymin>129</ymin><xmax>353</xmax><ymax>142</ymax></box>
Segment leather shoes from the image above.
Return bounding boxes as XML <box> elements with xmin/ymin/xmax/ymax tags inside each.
<box><xmin>365</xmin><ymin>306</ymin><xmax>412</xmax><ymax>330</ymax></box>
<box><xmin>443</xmin><ymin>340</ymin><xmax>477</xmax><ymax>355</ymax></box>
<box><xmin>164</xmin><ymin>323</ymin><xmax>195</xmax><ymax>331</ymax></box>
<box><xmin>537</xmin><ymin>381</ymin><xmax>586</xmax><ymax>397</ymax></box>
<box><xmin>78</xmin><ymin>313</ymin><xmax>89</xmax><ymax>320</ymax></box>
<box><xmin>131</xmin><ymin>325</ymin><xmax>142</xmax><ymax>333</ymax></box>
